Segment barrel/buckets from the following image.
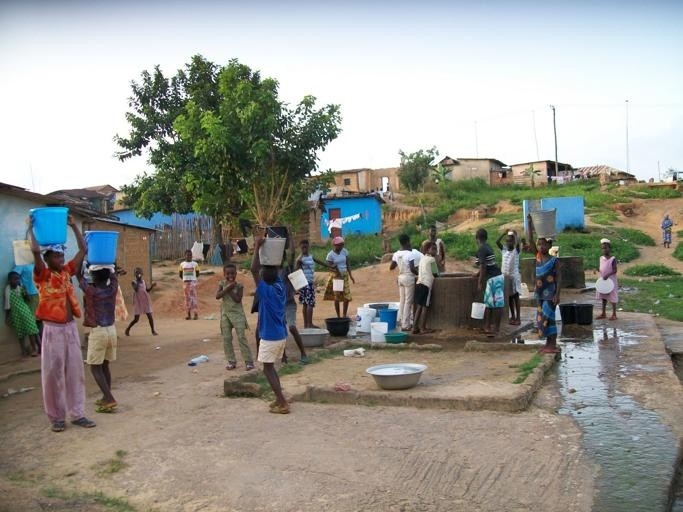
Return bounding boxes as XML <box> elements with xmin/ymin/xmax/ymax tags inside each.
<box><xmin>95</xmin><ymin>398</ymin><xmax>106</xmax><ymax>404</ymax></box>
<box><xmin>596</xmin><ymin>314</ymin><xmax>617</xmax><ymax>320</ymax></box>
<box><xmin>269</xmin><ymin>401</ymin><xmax>290</xmax><ymax>413</ymax></box>
<box><xmin>70</xmin><ymin>416</ymin><xmax>96</xmax><ymax>427</ymax></box>
<box><xmin>538</xmin><ymin>345</ymin><xmax>560</xmax><ymax>353</ymax></box>
<box><xmin>95</xmin><ymin>404</ymin><xmax>118</xmax><ymax>413</ymax></box>
<box><xmin>52</xmin><ymin>421</ymin><xmax>65</xmax><ymax>431</ymax></box>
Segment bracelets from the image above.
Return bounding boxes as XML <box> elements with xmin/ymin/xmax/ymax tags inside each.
<box><xmin>323</xmin><ymin>318</ymin><xmax>350</xmax><ymax>338</ymax></box>
<box><xmin>365</xmin><ymin>364</ymin><xmax>429</xmax><ymax>391</ymax></box>
<box><xmin>384</xmin><ymin>332</ymin><xmax>408</xmax><ymax>343</ymax></box>
<box><xmin>295</xmin><ymin>328</ymin><xmax>330</xmax><ymax>346</ymax></box>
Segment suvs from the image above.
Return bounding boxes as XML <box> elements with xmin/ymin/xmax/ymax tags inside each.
<box><xmin>29</xmin><ymin>207</ymin><xmax>68</xmax><ymax>246</ymax></box>
<box><xmin>324</xmin><ymin>318</ymin><xmax>351</xmax><ymax>337</ymax></box>
<box><xmin>288</xmin><ymin>269</ymin><xmax>308</xmax><ymax>291</ymax></box>
<box><xmin>258</xmin><ymin>238</ymin><xmax>287</xmax><ymax>266</ymax></box>
<box><xmin>85</xmin><ymin>230</ymin><xmax>119</xmax><ymax>264</ymax></box>
<box><xmin>548</xmin><ymin>246</ymin><xmax>559</xmax><ymax>258</ymax></box>
<box><xmin>411</xmin><ymin>249</ymin><xmax>424</xmax><ymax>268</ymax></box>
<box><xmin>368</xmin><ymin>304</ymin><xmax>389</xmax><ymax>322</ymax></box>
<box><xmin>356</xmin><ymin>307</ymin><xmax>377</xmax><ymax>332</ymax></box>
<box><xmin>576</xmin><ymin>304</ymin><xmax>592</xmax><ymax>325</ymax></box>
<box><xmin>519</xmin><ymin>283</ymin><xmax>529</xmax><ymax>298</ymax></box>
<box><xmin>559</xmin><ymin>304</ymin><xmax>576</xmax><ymax>324</ymax></box>
<box><xmin>378</xmin><ymin>309</ymin><xmax>398</xmax><ymax>330</ymax></box>
<box><xmin>530</xmin><ymin>208</ymin><xmax>556</xmax><ymax>238</ymax></box>
<box><xmin>371</xmin><ymin>322</ymin><xmax>388</xmax><ymax>343</ymax></box>
<box><xmin>471</xmin><ymin>302</ymin><xmax>486</xmax><ymax>320</ymax></box>
<box><xmin>333</xmin><ymin>279</ymin><xmax>344</xmax><ymax>292</ymax></box>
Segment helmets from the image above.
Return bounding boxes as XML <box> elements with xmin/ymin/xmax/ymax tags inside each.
<box><xmin>594</xmin><ymin>277</ymin><xmax>614</xmax><ymax>294</ymax></box>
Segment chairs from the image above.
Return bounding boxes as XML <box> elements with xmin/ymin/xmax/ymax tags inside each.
<box><xmin>246</xmin><ymin>363</ymin><xmax>253</xmax><ymax>370</ymax></box>
<box><xmin>225</xmin><ymin>362</ymin><xmax>236</xmax><ymax>369</ymax></box>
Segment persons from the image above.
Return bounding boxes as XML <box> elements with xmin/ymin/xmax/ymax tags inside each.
<box><xmin>474</xmin><ymin>227</ymin><xmax>503</xmax><ymax>334</ymax></box>
<box><xmin>526</xmin><ymin>212</ymin><xmax>560</xmax><ymax>356</ymax></box>
<box><xmin>25</xmin><ymin>216</ymin><xmax>96</xmax><ymax>432</ymax></box>
<box><xmin>497</xmin><ymin>229</ymin><xmax>522</xmax><ymax>326</ymax></box>
<box><xmin>177</xmin><ymin>248</ymin><xmax>201</xmax><ymax>319</ymax></box>
<box><xmin>250</xmin><ymin>236</ymin><xmax>291</xmax><ymax>414</ymax></box>
<box><xmin>594</xmin><ymin>236</ymin><xmax>620</xmax><ymax>321</ymax></box>
<box><xmin>74</xmin><ymin>266</ymin><xmax>117</xmax><ymax>414</ymax></box>
<box><xmin>280</xmin><ymin>232</ymin><xmax>307</xmax><ymax>363</ymax></box>
<box><xmin>660</xmin><ymin>215</ymin><xmax>673</xmax><ymax>248</ymax></box>
<box><xmin>323</xmin><ymin>237</ymin><xmax>356</xmax><ymax>321</ymax></box>
<box><xmin>10</xmin><ymin>264</ymin><xmax>37</xmax><ymax>355</ymax></box>
<box><xmin>125</xmin><ymin>267</ymin><xmax>158</xmax><ymax>338</ymax></box>
<box><xmin>391</xmin><ymin>224</ymin><xmax>445</xmax><ymax>336</ymax></box>
<box><xmin>293</xmin><ymin>240</ymin><xmax>331</xmax><ymax>327</ymax></box>
<box><xmin>214</xmin><ymin>263</ymin><xmax>252</xmax><ymax>370</ymax></box>
<box><xmin>4</xmin><ymin>272</ymin><xmax>38</xmax><ymax>357</ymax></box>
